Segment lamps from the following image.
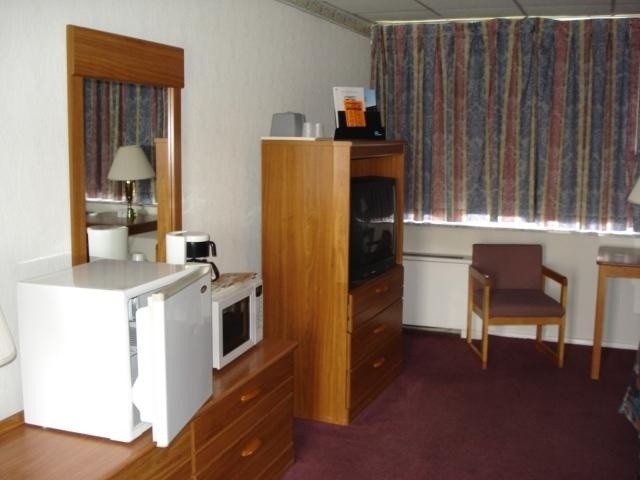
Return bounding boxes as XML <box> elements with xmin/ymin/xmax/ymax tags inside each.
<box><xmin>105</xmin><ymin>144</ymin><xmax>156</xmax><ymax>222</ymax></box>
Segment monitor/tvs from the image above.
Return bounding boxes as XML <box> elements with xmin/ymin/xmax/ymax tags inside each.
<box><xmin>349</xmin><ymin>176</ymin><xmax>396</xmax><ymax>291</ymax></box>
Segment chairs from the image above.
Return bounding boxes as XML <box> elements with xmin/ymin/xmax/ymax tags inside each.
<box><xmin>465</xmin><ymin>242</ymin><xmax>570</xmax><ymax>373</ymax></box>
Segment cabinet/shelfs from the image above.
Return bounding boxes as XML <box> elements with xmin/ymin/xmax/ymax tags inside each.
<box><xmin>259</xmin><ymin>138</ymin><xmax>407</xmax><ymax>427</ymax></box>
<box><xmin>0</xmin><ymin>339</ymin><xmax>304</xmax><ymax>480</ymax></box>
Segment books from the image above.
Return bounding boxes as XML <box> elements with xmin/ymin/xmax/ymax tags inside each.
<box><xmin>332</xmin><ymin>83</ymin><xmax>382</xmax><ymax>133</ymax></box>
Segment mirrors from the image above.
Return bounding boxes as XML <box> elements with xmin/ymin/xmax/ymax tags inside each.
<box><xmin>66</xmin><ymin>23</ymin><xmax>185</xmax><ymax>267</ymax></box>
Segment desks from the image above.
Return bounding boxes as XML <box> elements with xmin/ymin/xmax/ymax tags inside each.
<box><xmin>590</xmin><ymin>246</ymin><xmax>640</xmax><ymax>381</ymax></box>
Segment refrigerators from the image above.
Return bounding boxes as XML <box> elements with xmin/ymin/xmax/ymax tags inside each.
<box><xmin>17</xmin><ymin>257</ymin><xmax>213</xmax><ymax>449</ymax></box>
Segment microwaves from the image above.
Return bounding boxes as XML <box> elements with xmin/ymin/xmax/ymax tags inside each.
<box><xmin>212</xmin><ymin>276</ymin><xmax>264</xmax><ymax>371</ymax></box>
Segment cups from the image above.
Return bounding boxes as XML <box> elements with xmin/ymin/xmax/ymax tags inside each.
<box><xmin>301</xmin><ymin>122</ymin><xmax>324</xmax><ymax>138</ymax></box>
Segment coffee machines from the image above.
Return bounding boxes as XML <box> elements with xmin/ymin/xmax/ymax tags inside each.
<box><xmin>164</xmin><ymin>230</ymin><xmax>219</xmax><ymax>285</ymax></box>
